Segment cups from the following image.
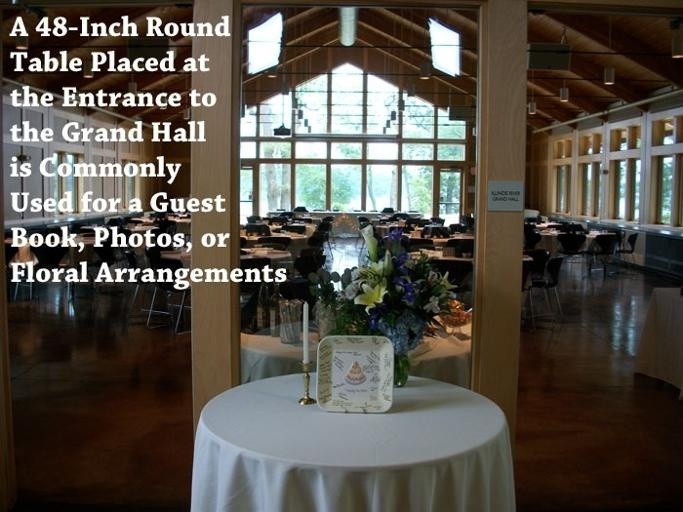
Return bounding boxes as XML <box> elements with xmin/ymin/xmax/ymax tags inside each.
<box><xmin>277</xmin><ymin>299</ymin><xmax>303</xmax><ymax>345</ymax></box>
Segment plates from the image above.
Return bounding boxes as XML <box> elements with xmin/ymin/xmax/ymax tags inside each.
<box><xmin>315</xmin><ymin>333</ymin><xmax>395</xmax><ymax>415</ymax></box>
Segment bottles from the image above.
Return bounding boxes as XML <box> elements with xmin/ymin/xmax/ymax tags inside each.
<box><xmin>254</xmin><ymin>282</ymin><xmax>268</xmax><ymax>330</ymax></box>
<box><xmin>268</xmin><ymin>281</ymin><xmax>285</xmax><ymax>337</ymax></box>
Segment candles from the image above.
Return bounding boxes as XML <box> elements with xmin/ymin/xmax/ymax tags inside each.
<box><xmin>301</xmin><ymin>301</ymin><xmax>310</xmax><ymax>364</ymax></box>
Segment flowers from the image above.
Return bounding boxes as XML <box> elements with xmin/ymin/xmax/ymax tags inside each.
<box><xmin>338</xmin><ymin>245</ymin><xmax>457</xmax><ymax>351</ymax></box>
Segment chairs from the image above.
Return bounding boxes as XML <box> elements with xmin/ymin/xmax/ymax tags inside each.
<box><xmin>354</xmin><ymin>207</ymin><xmax>638</xmax><ymax>331</ymax></box>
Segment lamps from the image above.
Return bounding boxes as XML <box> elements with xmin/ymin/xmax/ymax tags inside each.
<box><xmin>380</xmin><ymin>0</ymin><xmax>434</xmax><ymax>135</ymax></box>
<box><xmin>272</xmin><ymin>0</ymin><xmax>312</xmax><ymax>141</ymax></box>
<box><xmin>528</xmin><ymin>17</ymin><xmax>617</xmax><ymax>115</ymax></box>
